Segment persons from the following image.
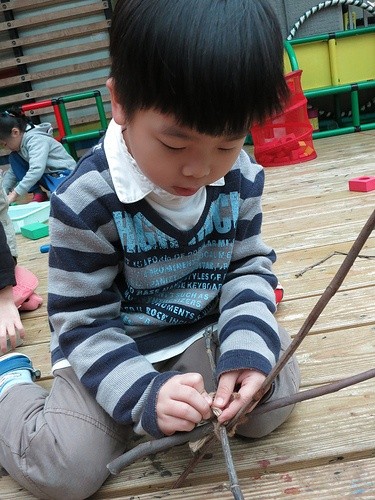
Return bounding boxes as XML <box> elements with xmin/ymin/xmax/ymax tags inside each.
<box><xmin>0</xmin><ymin>105</ymin><xmax>78</xmax><ymax>202</ymax></box>
<box><xmin>0</xmin><ymin>176</ymin><xmax>42</xmax><ymax>353</ymax></box>
<box><xmin>0</xmin><ymin>0</ymin><xmax>301</xmax><ymax>500</ymax></box>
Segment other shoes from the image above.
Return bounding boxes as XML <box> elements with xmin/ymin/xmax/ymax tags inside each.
<box><xmin>31</xmin><ymin>191</ymin><xmax>49</xmax><ymax>202</ymax></box>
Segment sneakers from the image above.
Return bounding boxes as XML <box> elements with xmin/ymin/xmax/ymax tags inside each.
<box><xmin>0</xmin><ymin>352</ymin><xmax>41</xmax><ymax>379</ymax></box>
